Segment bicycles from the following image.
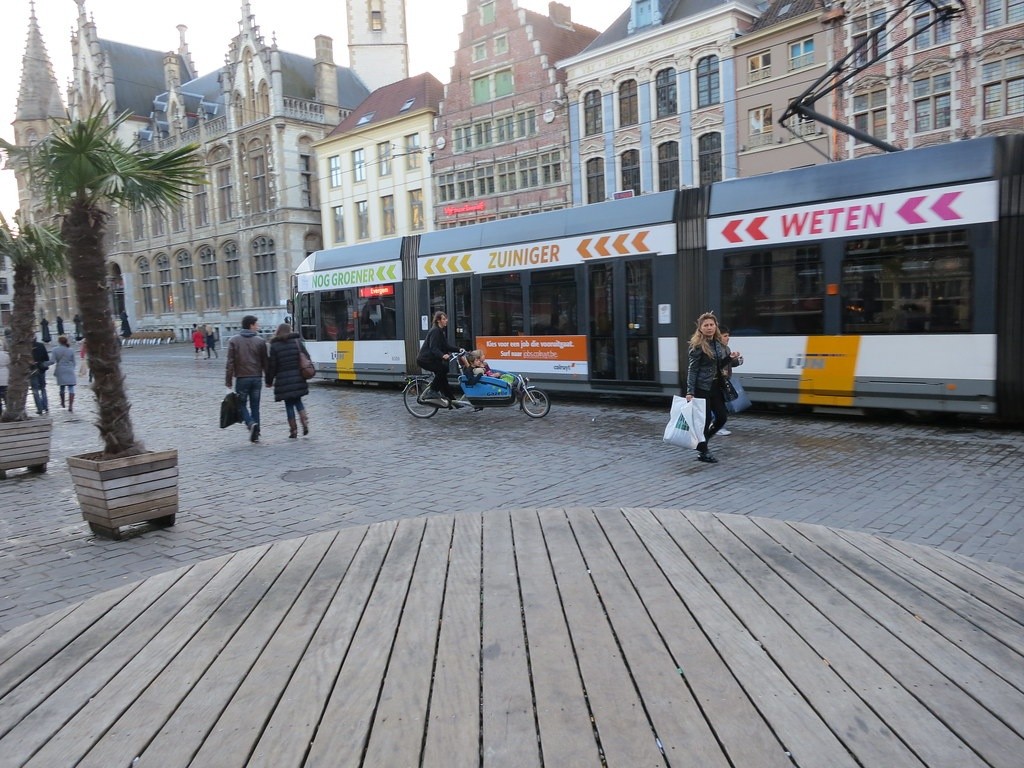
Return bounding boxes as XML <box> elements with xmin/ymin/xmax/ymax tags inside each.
<box><xmin>402</xmin><ymin>348</ymin><xmax>550</xmax><ymax>418</ymax></box>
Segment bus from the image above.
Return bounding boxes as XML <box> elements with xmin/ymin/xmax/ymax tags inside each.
<box><xmin>285</xmin><ymin>0</ymin><xmax>1024</xmax><ymax>431</ymax></box>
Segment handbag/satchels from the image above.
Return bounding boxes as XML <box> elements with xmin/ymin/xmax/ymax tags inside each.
<box><xmin>220</xmin><ymin>389</ymin><xmax>243</xmax><ymax>428</ymax></box>
<box><xmin>725</xmin><ymin>374</ymin><xmax>752</xmax><ymax>415</ymax></box>
<box><xmin>293</xmin><ymin>337</ymin><xmax>316</xmax><ymax>379</ymax></box>
<box><xmin>663</xmin><ymin>394</ymin><xmax>706</xmax><ymax>450</ymax></box>
<box><xmin>79</xmin><ymin>359</ymin><xmax>89</xmax><ymax>378</ymax></box>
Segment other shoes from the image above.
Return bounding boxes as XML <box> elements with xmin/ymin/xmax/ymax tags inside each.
<box><xmin>426</xmin><ymin>389</ymin><xmax>441</xmax><ymax>399</ymax></box>
<box><xmin>449</xmin><ymin>400</ymin><xmax>464</xmax><ymax>410</ymax></box>
<box><xmin>35</xmin><ymin>408</ymin><xmax>43</xmax><ymax>414</ymax></box>
<box><xmin>249</xmin><ymin>423</ymin><xmax>262</xmax><ymax>442</ymax></box>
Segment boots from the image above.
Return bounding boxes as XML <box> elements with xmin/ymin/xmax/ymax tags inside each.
<box><xmin>194</xmin><ymin>352</ymin><xmax>199</xmax><ymax>360</ymax></box>
<box><xmin>708</xmin><ymin>424</ymin><xmax>719</xmax><ymax>440</ymax></box>
<box><xmin>298</xmin><ymin>410</ymin><xmax>309</xmax><ymax>435</ymax></box>
<box><xmin>60</xmin><ymin>391</ymin><xmax>65</xmax><ymax>408</ymax></box>
<box><xmin>202</xmin><ymin>351</ymin><xmax>206</xmax><ymax>359</ymax></box>
<box><xmin>68</xmin><ymin>393</ymin><xmax>74</xmax><ymax>412</ymax></box>
<box><xmin>698</xmin><ymin>442</ymin><xmax>718</xmax><ymax>463</ymax></box>
<box><xmin>288</xmin><ymin>415</ymin><xmax>297</xmax><ymax>438</ymax></box>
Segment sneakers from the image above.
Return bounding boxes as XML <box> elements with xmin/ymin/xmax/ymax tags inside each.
<box><xmin>716</xmin><ymin>427</ymin><xmax>731</xmax><ymax>436</ymax></box>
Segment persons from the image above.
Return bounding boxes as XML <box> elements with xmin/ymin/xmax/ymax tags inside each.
<box><xmin>686</xmin><ymin>312</ymin><xmax>740</xmax><ymax>463</ymax></box>
<box><xmin>42</xmin><ymin>335</ymin><xmax>77</xmax><ymax>411</ymax></box>
<box><xmin>496</xmin><ymin>321</ymin><xmax>508</xmax><ymax>335</ymax></box>
<box><xmin>27</xmin><ymin>330</ymin><xmax>49</xmax><ymax>414</ymax></box>
<box><xmin>464</xmin><ymin>350</ymin><xmax>529</xmax><ymax>388</ymax></box>
<box><xmin>708</xmin><ymin>325</ymin><xmax>743</xmax><ymax>435</ymax></box>
<box><xmin>73</xmin><ymin>314</ymin><xmax>84</xmax><ymax>337</ymax></box>
<box><xmin>265</xmin><ymin>324</ymin><xmax>312</xmax><ymax>439</ymax></box>
<box><xmin>80</xmin><ymin>337</ymin><xmax>96</xmax><ymax>383</ymax></box>
<box><xmin>57</xmin><ymin>316</ymin><xmax>64</xmax><ymax>335</ymax></box>
<box><xmin>192</xmin><ymin>327</ymin><xmax>206</xmax><ymax>360</ymax></box>
<box><xmin>416</xmin><ymin>311</ymin><xmax>469</xmax><ymax>409</ymax></box>
<box><xmin>205</xmin><ymin>325</ymin><xmax>219</xmax><ymax>359</ymax></box>
<box><xmin>191</xmin><ymin>323</ymin><xmax>198</xmax><ymax>339</ymax></box>
<box><xmin>226</xmin><ymin>315</ymin><xmax>271</xmax><ymax>442</ymax></box>
<box><xmin>3</xmin><ymin>327</ymin><xmax>12</xmax><ymax>351</ymax></box>
<box><xmin>40</xmin><ymin>318</ymin><xmax>51</xmax><ymax>343</ymax></box>
<box><xmin>119</xmin><ymin>309</ymin><xmax>132</xmax><ymax>338</ymax></box>
<box><xmin>0</xmin><ymin>339</ymin><xmax>12</xmax><ymax>419</ymax></box>
